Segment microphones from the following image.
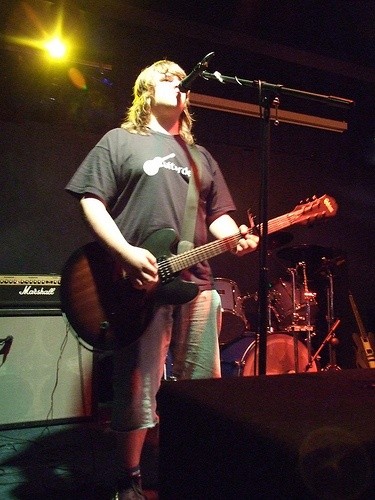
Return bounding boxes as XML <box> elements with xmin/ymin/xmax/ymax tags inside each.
<box><xmin>179</xmin><ymin>52</ymin><xmax>218</xmax><ymax>93</ymax></box>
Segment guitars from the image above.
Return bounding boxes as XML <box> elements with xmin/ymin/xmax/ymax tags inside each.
<box><xmin>59</xmin><ymin>192</ymin><xmax>339</xmax><ymax>353</ymax></box>
<box><xmin>347</xmin><ymin>289</ymin><xmax>375</xmax><ymax>370</ymax></box>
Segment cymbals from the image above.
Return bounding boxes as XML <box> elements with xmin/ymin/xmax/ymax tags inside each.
<box><xmin>268</xmin><ymin>244</ymin><xmax>337</xmax><ymax>261</ymax></box>
<box><xmin>254</xmin><ymin>231</ymin><xmax>295</xmax><ymax>250</ymax></box>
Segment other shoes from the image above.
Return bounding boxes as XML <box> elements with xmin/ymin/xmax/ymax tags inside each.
<box><xmin>118</xmin><ymin>475</ymin><xmax>146</xmax><ymax>500</ymax></box>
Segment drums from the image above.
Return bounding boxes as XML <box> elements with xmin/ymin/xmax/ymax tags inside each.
<box><xmin>210</xmin><ymin>276</ymin><xmax>248</xmax><ymax>347</ymax></box>
<box><xmin>219</xmin><ymin>330</ymin><xmax>319</xmax><ymax>377</ymax></box>
<box><xmin>261</xmin><ymin>278</ymin><xmax>322</xmax><ymax>334</ymax></box>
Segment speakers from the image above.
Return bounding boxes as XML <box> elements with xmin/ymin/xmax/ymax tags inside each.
<box><xmin>155</xmin><ymin>367</ymin><xmax>375</xmax><ymax>500</ymax></box>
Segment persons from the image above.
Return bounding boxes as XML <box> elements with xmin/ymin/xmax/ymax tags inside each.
<box><xmin>64</xmin><ymin>58</ymin><xmax>261</xmax><ymax>500</ymax></box>
